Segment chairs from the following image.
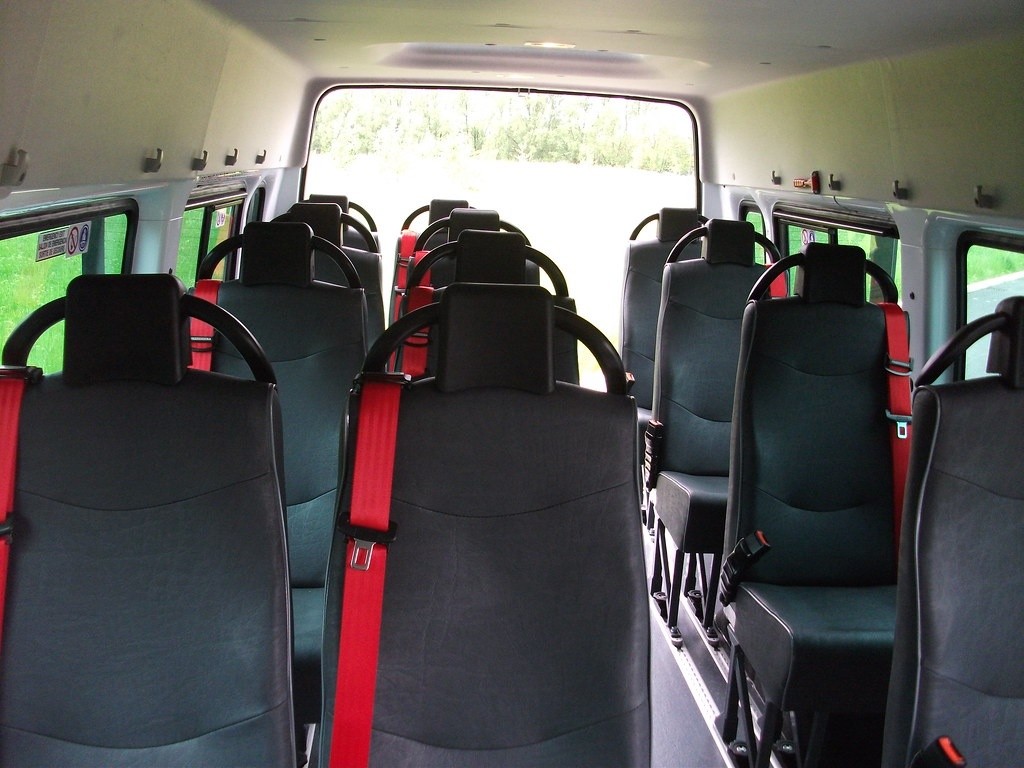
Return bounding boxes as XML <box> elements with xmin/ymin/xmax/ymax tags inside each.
<box><xmin>616</xmin><ymin>207</ymin><xmax>710</xmax><ymax>521</ymax></box>
<box><xmin>406</xmin><ymin>209</ymin><xmax>540</xmax><ymax>290</ymax></box>
<box><xmin>387</xmin><ymin>198</ymin><xmax>478</xmax><ymax>325</ymax></box>
<box><xmin>186</xmin><ymin>220</ymin><xmax>367</xmax><ymax>723</ymax></box>
<box><xmin>641</xmin><ymin>219</ymin><xmax>790</xmax><ymax>656</ymax></box>
<box><xmin>274</xmin><ymin>202</ymin><xmax>386</xmax><ymax>352</ymax></box>
<box><xmin>882</xmin><ymin>298</ymin><xmax>1024</xmax><ymax>768</ymax></box>
<box><xmin>712</xmin><ymin>249</ymin><xmax>911</xmax><ymax>768</ymax></box>
<box><xmin>302</xmin><ymin>279</ymin><xmax>652</xmax><ymax>768</ymax></box>
<box><xmin>302</xmin><ymin>193</ymin><xmax>380</xmax><ymax>254</ymax></box>
<box><xmin>393</xmin><ymin>230</ymin><xmax>580</xmax><ymax>386</ymax></box>
<box><xmin>0</xmin><ymin>275</ymin><xmax>299</xmax><ymax>768</ymax></box>
